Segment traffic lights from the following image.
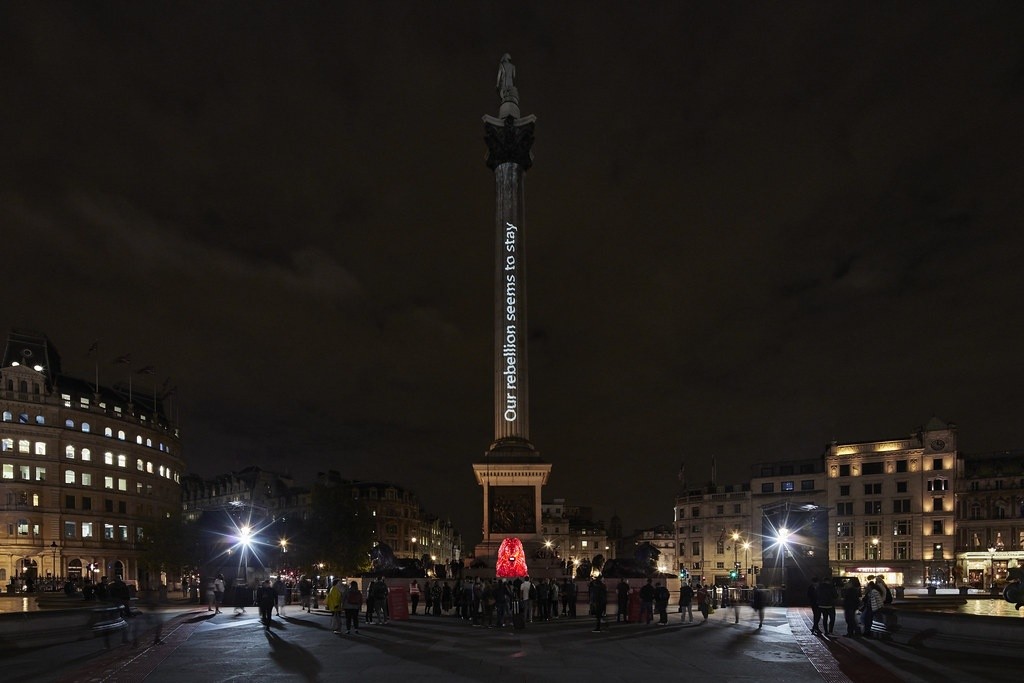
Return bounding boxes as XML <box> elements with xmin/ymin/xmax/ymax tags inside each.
<box><xmin>754</xmin><ymin>566</ymin><xmax>758</xmax><ymax>574</ymax></box>
<box><xmin>730</xmin><ymin>571</ymin><xmax>737</xmax><ymax>578</ymax></box>
<box><xmin>681</xmin><ymin>572</ymin><xmax>684</xmax><ymax>578</ymax></box>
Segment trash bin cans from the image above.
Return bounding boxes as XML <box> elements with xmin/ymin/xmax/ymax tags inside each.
<box><xmin>990</xmin><ymin>588</ymin><xmax>999</xmax><ymax>595</ymax></box>
<box><xmin>895</xmin><ymin>586</ymin><xmax>905</xmax><ymax>599</ymax></box>
<box><xmin>927</xmin><ymin>586</ymin><xmax>937</xmax><ymax>595</ymax></box>
<box><xmin>958</xmin><ymin>586</ymin><xmax>968</xmax><ymax>595</ymax></box>
<box><xmin>127</xmin><ymin>584</ymin><xmax>136</xmax><ymax>598</ymax></box>
<box><xmin>6</xmin><ymin>584</ymin><xmax>15</xmax><ymax>596</ymax></box>
<box><xmin>189</xmin><ymin>588</ymin><xmax>197</xmax><ymax>598</ymax></box>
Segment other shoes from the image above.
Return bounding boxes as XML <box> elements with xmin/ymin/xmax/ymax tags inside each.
<box><xmin>657</xmin><ymin>620</ymin><xmax>668</xmax><ymax>626</ymax></box>
<box><xmin>843</xmin><ymin>634</ymin><xmax>852</xmax><ymax>638</ymax></box>
<box><xmin>828</xmin><ymin>632</ymin><xmax>837</xmax><ymax>638</ymax></box>
<box><xmin>334</xmin><ymin>630</ymin><xmax>342</xmax><ymax>634</ymax></box>
<box><xmin>355</xmin><ymin>629</ymin><xmax>359</xmax><ymax>634</ymax></box>
<box><xmin>345</xmin><ymin>630</ymin><xmax>350</xmax><ymax>634</ymax></box>
<box><xmin>591</xmin><ymin>629</ymin><xmax>600</xmax><ymax>633</ymax></box>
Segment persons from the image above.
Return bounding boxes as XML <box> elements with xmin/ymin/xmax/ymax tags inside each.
<box><xmin>696</xmin><ymin>583</ymin><xmax>710</xmax><ymax>624</ymax></box>
<box><xmin>257</xmin><ymin>576</ymin><xmax>287</xmax><ymax>630</ymax></box>
<box><xmin>654</xmin><ymin>583</ymin><xmax>671</xmax><ymax>625</ymax></box>
<box><xmin>588</xmin><ymin>576</ymin><xmax>607</xmax><ymax>633</ymax></box>
<box><xmin>64</xmin><ymin>578</ymin><xmax>79</xmax><ymax>596</ymax></box>
<box><xmin>299</xmin><ymin>575</ymin><xmax>311</xmax><ymax>613</ymax></box>
<box><xmin>214</xmin><ymin>574</ymin><xmax>224</xmax><ymax>614</ymax></box>
<box><xmin>94</xmin><ymin>576</ymin><xmax>130</xmax><ymax>615</ymax></box>
<box><xmin>441</xmin><ymin>582</ymin><xmax>453</xmax><ymax>615</ymax></box>
<box><xmin>496</xmin><ymin>52</ymin><xmax>516</xmax><ymax>99</ymax></box>
<box><xmin>432</xmin><ymin>581</ymin><xmax>442</xmax><ymax>616</ymax></box>
<box><xmin>453</xmin><ymin>576</ymin><xmax>577</xmax><ymax>629</ymax></box>
<box><xmin>424</xmin><ymin>580</ymin><xmax>432</xmax><ymax>615</ymax></box>
<box><xmin>560</xmin><ymin>557</ymin><xmax>574</xmax><ymax>575</ymax></box>
<box><xmin>323</xmin><ymin>578</ymin><xmax>363</xmax><ymax>634</ymax></box>
<box><xmin>365</xmin><ymin>576</ymin><xmax>390</xmax><ymax>624</ymax></box>
<box><xmin>409</xmin><ymin>580</ymin><xmax>422</xmax><ymax>616</ymax></box>
<box><xmin>576</xmin><ymin>558</ymin><xmax>592</xmax><ymax>581</ymax></box>
<box><xmin>445</xmin><ymin>558</ymin><xmax>464</xmax><ymax>579</ymax></box>
<box><xmin>808</xmin><ymin>576</ymin><xmax>839</xmax><ymax>636</ymax></box>
<box><xmin>207</xmin><ymin>577</ymin><xmax>215</xmax><ymax>611</ymax></box>
<box><xmin>678</xmin><ymin>582</ymin><xmax>694</xmax><ymax>624</ymax></box>
<box><xmin>616</xmin><ymin>577</ymin><xmax>630</xmax><ymax>622</ymax></box>
<box><xmin>753</xmin><ymin>586</ymin><xmax>766</xmax><ymax>630</ymax></box>
<box><xmin>637</xmin><ymin>578</ymin><xmax>655</xmax><ymax>624</ymax></box>
<box><xmin>841</xmin><ymin>575</ymin><xmax>893</xmax><ymax>639</ymax></box>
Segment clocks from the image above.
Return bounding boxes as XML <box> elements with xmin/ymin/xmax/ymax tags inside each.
<box><xmin>931</xmin><ymin>440</ymin><xmax>944</xmax><ymax>449</ymax></box>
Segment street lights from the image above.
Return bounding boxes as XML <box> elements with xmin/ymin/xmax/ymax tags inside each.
<box><xmin>779</xmin><ymin>529</ymin><xmax>786</xmax><ymax>605</ymax></box>
<box><xmin>50</xmin><ymin>541</ymin><xmax>58</xmax><ymax>592</ymax></box>
<box><xmin>412</xmin><ymin>538</ymin><xmax>417</xmax><ymax>559</ymax></box>
<box><xmin>242</xmin><ymin>528</ymin><xmax>249</xmax><ymax>583</ymax></box>
<box><xmin>745</xmin><ymin>543</ymin><xmax>748</xmax><ymax>586</ymax></box>
<box><xmin>734</xmin><ymin>534</ymin><xmax>738</xmax><ymax>568</ymax></box>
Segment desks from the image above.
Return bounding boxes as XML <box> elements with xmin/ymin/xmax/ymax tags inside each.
<box><xmin>286</xmin><ymin>588</ymin><xmax>301</xmax><ymax>601</ymax></box>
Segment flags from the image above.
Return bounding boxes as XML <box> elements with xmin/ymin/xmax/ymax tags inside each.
<box><xmin>162</xmin><ymin>377</ymin><xmax>173</xmax><ymax>386</ymax></box>
<box><xmin>87</xmin><ymin>343</ymin><xmax>97</xmax><ymax>355</ymax></box>
<box><xmin>112</xmin><ymin>352</ymin><xmax>132</xmax><ymax>367</ymax></box>
<box><xmin>143</xmin><ymin>368</ymin><xmax>157</xmax><ymax>376</ymax></box>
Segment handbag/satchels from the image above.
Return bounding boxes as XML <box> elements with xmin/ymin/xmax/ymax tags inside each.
<box><xmin>707</xmin><ymin>604</ymin><xmax>715</xmax><ymax>615</ymax></box>
